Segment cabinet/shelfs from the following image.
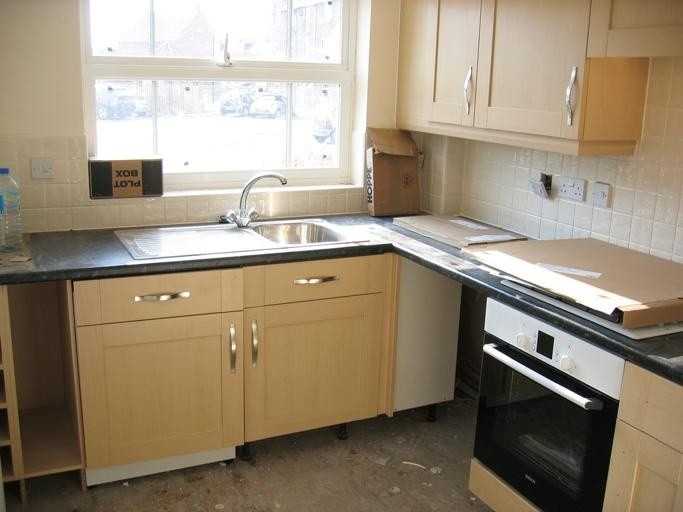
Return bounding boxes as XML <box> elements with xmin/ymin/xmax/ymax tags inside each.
<box><xmin>65</xmin><ymin>254</ymin><xmax>399</xmax><ymax>469</ymax></box>
<box><xmin>396</xmin><ymin>0</ymin><xmax>649</xmax><ymax>157</ymax></box>
<box><xmin>599</xmin><ymin>356</ymin><xmax>683</xmax><ymax>512</ymax></box>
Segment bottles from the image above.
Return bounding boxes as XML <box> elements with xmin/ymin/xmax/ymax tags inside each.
<box><xmin>0</xmin><ymin>168</ymin><xmax>22</xmax><ymax>252</ymax></box>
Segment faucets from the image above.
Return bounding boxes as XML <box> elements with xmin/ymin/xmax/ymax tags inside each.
<box><xmin>226</xmin><ymin>173</ymin><xmax>287</xmax><ymax>228</ymax></box>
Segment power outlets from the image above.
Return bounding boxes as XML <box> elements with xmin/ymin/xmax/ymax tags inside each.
<box><xmin>557</xmin><ymin>175</ymin><xmax>585</xmax><ymax>202</ymax></box>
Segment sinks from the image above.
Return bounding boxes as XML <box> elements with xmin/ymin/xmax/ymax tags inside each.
<box><xmin>252</xmin><ymin>218</ymin><xmax>369</xmax><ymax>250</ymax></box>
<box><xmin>112</xmin><ymin>216</ymin><xmax>259</xmax><ymax>260</ymax></box>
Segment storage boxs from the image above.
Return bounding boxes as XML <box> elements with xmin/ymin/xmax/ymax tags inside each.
<box><xmin>462</xmin><ymin>238</ymin><xmax>683</xmax><ymax>331</ymax></box>
<box><xmin>88</xmin><ymin>158</ymin><xmax>164</xmax><ymax>199</ymax></box>
<box><xmin>366</xmin><ymin>128</ymin><xmax>422</xmax><ymax>217</ymax></box>
